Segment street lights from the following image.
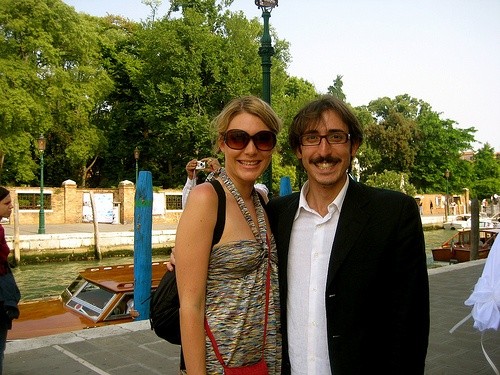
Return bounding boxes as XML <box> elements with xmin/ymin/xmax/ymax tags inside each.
<box><xmin>445</xmin><ymin>169</ymin><xmax>451</xmax><ymax>213</ymax></box>
<box><xmin>35</xmin><ymin>134</ymin><xmax>48</xmax><ymax>234</ymax></box>
<box><xmin>254</xmin><ymin>0</ymin><xmax>279</xmax><ymax>200</ymax></box>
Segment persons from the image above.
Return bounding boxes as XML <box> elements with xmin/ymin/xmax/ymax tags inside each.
<box><xmin>166</xmin><ymin>95</ymin><xmax>430</xmax><ymax>375</ymax></box>
<box><xmin>174</xmin><ymin>96</ymin><xmax>281</xmax><ymax>375</ymax></box>
<box><xmin>181</xmin><ymin>157</ymin><xmax>223</xmax><ymax>213</ymax></box>
<box><xmin>0</xmin><ymin>186</ymin><xmax>22</xmax><ymax>375</ymax></box>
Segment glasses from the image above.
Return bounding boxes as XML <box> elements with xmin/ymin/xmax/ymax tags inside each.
<box><xmin>299</xmin><ymin>132</ymin><xmax>350</xmax><ymax>146</ymax></box>
<box><xmin>221</xmin><ymin>129</ymin><xmax>277</xmax><ymax>151</ymax></box>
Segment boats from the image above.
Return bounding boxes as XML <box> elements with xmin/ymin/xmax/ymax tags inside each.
<box><xmin>443</xmin><ymin>214</ymin><xmax>491</xmax><ymax>230</ymax></box>
<box><xmin>7</xmin><ymin>260</ymin><xmax>169</xmax><ymax>341</ymax></box>
<box><xmin>431</xmin><ymin>228</ymin><xmax>499</xmax><ymax>263</ymax></box>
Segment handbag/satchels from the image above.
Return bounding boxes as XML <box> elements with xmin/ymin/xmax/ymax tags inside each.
<box><xmin>224</xmin><ymin>359</ymin><xmax>268</xmax><ymax>375</ymax></box>
<box><xmin>0</xmin><ymin>273</ymin><xmax>21</xmax><ymax>304</ymax></box>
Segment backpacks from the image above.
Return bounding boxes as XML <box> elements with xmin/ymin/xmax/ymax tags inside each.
<box><xmin>149</xmin><ymin>180</ymin><xmax>266</xmax><ymax>345</ymax></box>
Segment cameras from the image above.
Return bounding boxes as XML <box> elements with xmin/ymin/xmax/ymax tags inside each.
<box><xmin>195</xmin><ymin>161</ymin><xmax>206</xmax><ymax>169</ymax></box>
